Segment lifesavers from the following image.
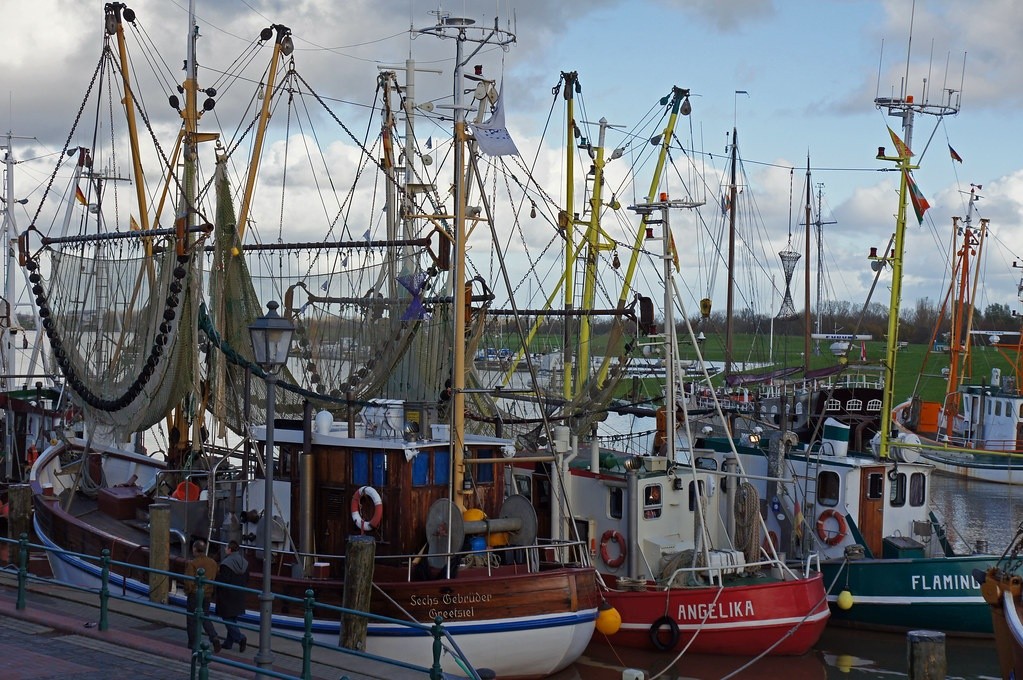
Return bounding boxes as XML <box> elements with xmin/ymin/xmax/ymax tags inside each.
<box><xmin>648</xmin><ymin>616</ymin><xmax>680</xmax><ymax>649</ymax></box>
<box><xmin>27</xmin><ymin>444</ymin><xmax>39</xmax><ymax>467</ymax></box>
<box><xmin>600</xmin><ymin>530</ymin><xmax>627</xmax><ymax>568</ymax></box>
<box><xmin>817</xmin><ymin>510</ymin><xmax>845</xmax><ymax>543</ymax></box>
<box><xmin>348</xmin><ymin>486</ymin><xmax>383</xmax><ymax>532</ymax></box>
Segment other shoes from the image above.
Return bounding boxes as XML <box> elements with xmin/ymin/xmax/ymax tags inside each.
<box><xmin>239</xmin><ymin>636</ymin><xmax>246</xmax><ymax>653</ymax></box>
<box><xmin>213</xmin><ymin>639</ymin><xmax>221</xmax><ymax>653</ymax></box>
<box><xmin>219</xmin><ymin>642</ymin><xmax>232</xmax><ymax>650</ymax></box>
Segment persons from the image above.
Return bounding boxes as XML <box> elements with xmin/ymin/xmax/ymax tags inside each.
<box><xmin>184</xmin><ymin>540</ymin><xmax>223</xmax><ymax>654</ymax></box>
<box><xmin>211</xmin><ymin>540</ymin><xmax>250</xmax><ymax>652</ymax></box>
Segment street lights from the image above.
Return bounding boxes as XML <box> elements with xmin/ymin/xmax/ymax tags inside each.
<box><xmin>247</xmin><ymin>299</ymin><xmax>297</xmax><ymax>663</ymax></box>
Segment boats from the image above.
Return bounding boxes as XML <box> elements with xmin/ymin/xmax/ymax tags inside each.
<box><xmin>30</xmin><ymin>1</ymin><xmax>599</xmax><ymax>676</ymax></box>
<box><xmin>685</xmin><ymin>0</ymin><xmax>1022</xmax><ymax>636</ymax></box>
<box><xmin>377</xmin><ymin>15</ymin><xmax>831</xmax><ymax>653</ymax></box>
<box><xmin>890</xmin><ymin>184</ymin><xmax>1023</xmax><ymax>484</ymax></box>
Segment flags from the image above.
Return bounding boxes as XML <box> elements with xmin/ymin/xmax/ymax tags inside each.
<box><xmin>948</xmin><ymin>145</ymin><xmax>962</xmax><ymax>164</ymax></box>
<box><xmin>888</xmin><ymin>126</ymin><xmax>916</xmax><ymax>158</ymax></box>
<box><xmin>904</xmin><ymin>170</ymin><xmax>931</xmax><ymax>228</ymax></box>
<box><xmin>860</xmin><ymin>341</ymin><xmax>867</xmax><ymax>361</ymax></box>
<box><xmin>469</xmin><ymin>79</ymin><xmax>519</xmax><ymax>156</ymax></box>
